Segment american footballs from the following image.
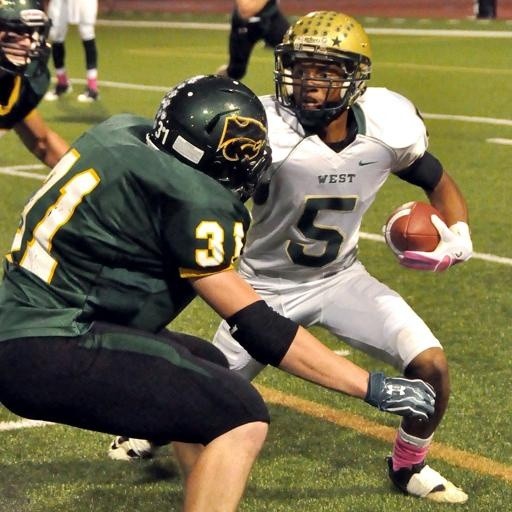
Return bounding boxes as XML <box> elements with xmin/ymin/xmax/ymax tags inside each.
<box><xmin>383</xmin><ymin>201</ymin><xmax>446</xmax><ymax>257</ymax></box>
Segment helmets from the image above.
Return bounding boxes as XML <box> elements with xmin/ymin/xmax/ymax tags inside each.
<box><xmin>144</xmin><ymin>72</ymin><xmax>273</xmax><ymax>205</ymax></box>
<box><xmin>0</xmin><ymin>0</ymin><xmax>53</xmax><ymax>78</ymax></box>
<box><xmin>271</xmin><ymin>9</ymin><xmax>374</xmax><ymax>127</ymax></box>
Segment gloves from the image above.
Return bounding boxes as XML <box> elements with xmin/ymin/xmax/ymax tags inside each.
<box><xmin>363</xmin><ymin>369</ymin><xmax>437</xmax><ymax>423</ymax></box>
<box><xmin>398</xmin><ymin>213</ymin><xmax>474</xmax><ymax>274</ymax></box>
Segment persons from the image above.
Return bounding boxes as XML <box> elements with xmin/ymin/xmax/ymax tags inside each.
<box><xmin>0</xmin><ymin>0</ymin><xmax>71</xmax><ymax>171</ymax></box>
<box><xmin>216</xmin><ymin>0</ymin><xmax>291</xmax><ymax>81</ymax></box>
<box><xmin>472</xmin><ymin>0</ymin><xmax>496</xmax><ymax>20</ymax></box>
<box><xmin>104</xmin><ymin>10</ymin><xmax>475</xmax><ymax>505</ymax></box>
<box><xmin>0</xmin><ymin>73</ymin><xmax>439</xmax><ymax>511</ymax></box>
<box><xmin>44</xmin><ymin>0</ymin><xmax>100</xmax><ymax>104</ymax></box>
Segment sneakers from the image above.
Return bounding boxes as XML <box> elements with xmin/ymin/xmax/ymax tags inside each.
<box><xmin>44</xmin><ymin>83</ymin><xmax>72</xmax><ymax>102</ymax></box>
<box><xmin>78</xmin><ymin>88</ymin><xmax>100</xmax><ymax>102</ymax></box>
<box><xmin>385</xmin><ymin>455</ymin><xmax>468</xmax><ymax>504</ymax></box>
<box><xmin>109</xmin><ymin>435</ymin><xmax>157</xmax><ymax>461</ymax></box>
